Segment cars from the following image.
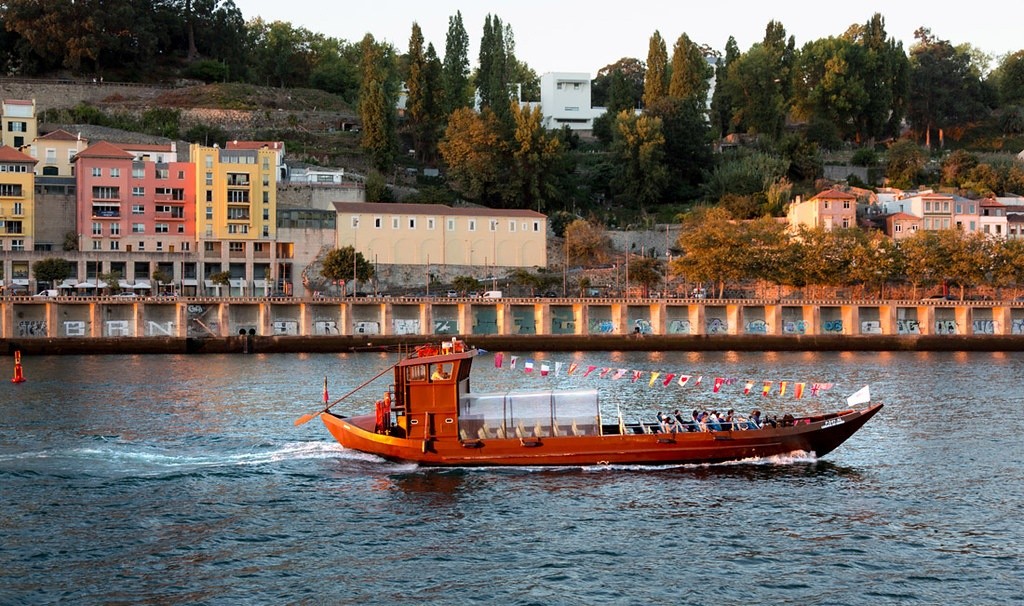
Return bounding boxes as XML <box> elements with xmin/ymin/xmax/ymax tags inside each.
<box><xmin>110</xmin><ymin>292</ymin><xmax>143</xmax><ymax>300</ymax></box>
<box><xmin>313</xmin><ymin>291</ymin><xmax>324</xmax><ymax>302</ymax></box>
<box><xmin>148</xmin><ymin>292</ymin><xmax>177</xmax><ymax>301</ymax></box>
<box><xmin>544</xmin><ymin>291</ymin><xmax>557</xmax><ymax>298</ymax></box>
<box><xmin>263</xmin><ymin>292</ymin><xmax>289</xmax><ymax>301</ymax></box>
<box><xmin>400</xmin><ymin>289</ymin><xmax>502</xmax><ymax>301</ymax></box>
<box><xmin>345</xmin><ymin>292</ymin><xmax>367</xmax><ymax>300</ymax></box>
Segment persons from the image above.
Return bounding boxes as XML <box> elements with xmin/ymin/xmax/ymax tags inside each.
<box><xmin>656</xmin><ymin>409</ymin><xmax>792</xmax><ymax>433</ymax></box>
<box><xmin>431</xmin><ymin>364</ymin><xmax>450</xmax><ymax>380</ymax></box>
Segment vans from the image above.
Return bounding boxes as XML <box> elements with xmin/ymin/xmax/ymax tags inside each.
<box><xmin>33</xmin><ymin>289</ymin><xmax>60</xmax><ymax>301</ymax></box>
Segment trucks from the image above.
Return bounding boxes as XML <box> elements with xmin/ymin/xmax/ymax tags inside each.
<box><xmin>693</xmin><ymin>288</ymin><xmax>706</xmax><ymax>299</ymax></box>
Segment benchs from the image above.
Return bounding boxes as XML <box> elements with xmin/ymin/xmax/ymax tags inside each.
<box><xmin>618</xmin><ymin>412</ymin><xmax>761</xmax><ymax>434</ymax></box>
<box><xmin>461</xmin><ymin>415</ymin><xmax>603</xmax><ymax>440</ymax></box>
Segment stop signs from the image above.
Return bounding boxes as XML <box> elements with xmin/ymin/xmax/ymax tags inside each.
<box><xmin>340</xmin><ymin>280</ymin><xmax>345</xmax><ymax>286</ymax></box>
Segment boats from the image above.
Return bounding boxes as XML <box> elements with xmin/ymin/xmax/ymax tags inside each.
<box><xmin>315</xmin><ymin>333</ymin><xmax>887</xmax><ymax>469</ymax></box>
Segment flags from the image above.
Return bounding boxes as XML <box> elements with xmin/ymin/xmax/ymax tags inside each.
<box><xmin>474</xmin><ymin>348</ymin><xmax>823</xmax><ymax>399</ymax></box>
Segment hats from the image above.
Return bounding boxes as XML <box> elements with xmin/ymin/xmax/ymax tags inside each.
<box><xmin>662</xmin><ymin>415</ymin><xmax>667</xmax><ymax>420</ymax></box>
